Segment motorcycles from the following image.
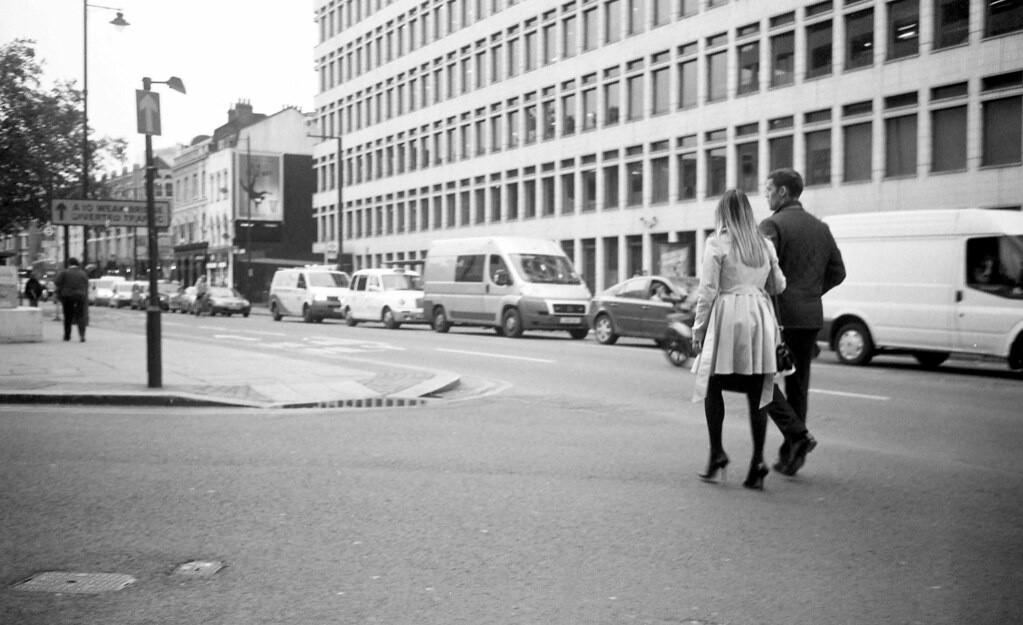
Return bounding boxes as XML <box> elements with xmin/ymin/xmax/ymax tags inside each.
<box><xmin>661</xmin><ymin>300</ymin><xmax>705</xmax><ymax>367</ymax></box>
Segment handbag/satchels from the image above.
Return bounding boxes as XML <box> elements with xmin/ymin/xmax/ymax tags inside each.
<box><xmin>777</xmin><ymin>343</ymin><xmax>796</xmax><ymax>376</ymax></box>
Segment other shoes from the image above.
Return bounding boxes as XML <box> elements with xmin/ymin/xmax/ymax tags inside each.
<box><xmin>774</xmin><ymin>462</ymin><xmax>795</xmax><ymax>478</ymax></box>
<box><xmin>783</xmin><ymin>432</ymin><xmax>817</xmax><ymax>475</ymax></box>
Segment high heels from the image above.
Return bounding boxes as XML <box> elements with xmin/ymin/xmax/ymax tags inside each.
<box><xmin>698</xmin><ymin>453</ymin><xmax>730</xmax><ymax>483</ymax></box>
<box><xmin>742</xmin><ymin>463</ymin><xmax>768</xmax><ymax>492</ymax></box>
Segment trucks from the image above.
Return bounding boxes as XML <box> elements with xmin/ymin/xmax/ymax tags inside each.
<box><xmin>812</xmin><ymin>210</ymin><xmax>1023</xmax><ymax>371</ymax></box>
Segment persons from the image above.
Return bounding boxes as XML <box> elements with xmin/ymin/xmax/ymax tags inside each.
<box><xmin>53</xmin><ymin>256</ymin><xmax>88</xmax><ymax>343</ymax></box>
<box><xmin>24</xmin><ymin>273</ymin><xmax>42</xmax><ymax>308</ymax></box>
<box><xmin>689</xmin><ymin>190</ymin><xmax>789</xmax><ymax>492</ymax></box>
<box><xmin>755</xmin><ymin>168</ymin><xmax>847</xmax><ymax>477</ymax></box>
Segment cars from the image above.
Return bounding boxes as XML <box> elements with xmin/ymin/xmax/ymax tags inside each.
<box><xmin>19</xmin><ymin>273</ymin><xmax>251</xmax><ymax>317</ymax></box>
<box><xmin>585</xmin><ymin>275</ymin><xmax>701</xmax><ymax>348</ymax></box>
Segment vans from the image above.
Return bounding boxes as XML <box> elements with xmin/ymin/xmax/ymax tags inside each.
<box><xmin>421</xmin><ymin>238</ymin><xmax>592</xmax><ymax>340</ymax></box>
<box><xmin>342</xmin><ymin>267</ymin><xmax>425</xmax><ymax>328</ymax></box>
<box><xmin>269</xmin><ymin>267</ymin><xmax>349</xmax><ymax>322</ymax></box>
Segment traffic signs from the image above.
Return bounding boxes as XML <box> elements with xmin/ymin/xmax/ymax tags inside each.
<box><xmin>52</xmin><ymin>199</ymin><xmax>170</xmax><ymax>227</ymax></box>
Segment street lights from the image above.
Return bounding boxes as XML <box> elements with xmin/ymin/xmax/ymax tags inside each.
<box><xmin>135</xmin><ymin>77</ymin><xmax>187</xmax><ymax>388</ymax></box>
<box><xmin>306</xmin><ymin>132</ymin><xmax>344</xmax><ymax>267</ymax></box>
<box><xmin>80</xmin><ymin>0</ymin><xmax>130</xmax><ymax>261</ymax></box>
